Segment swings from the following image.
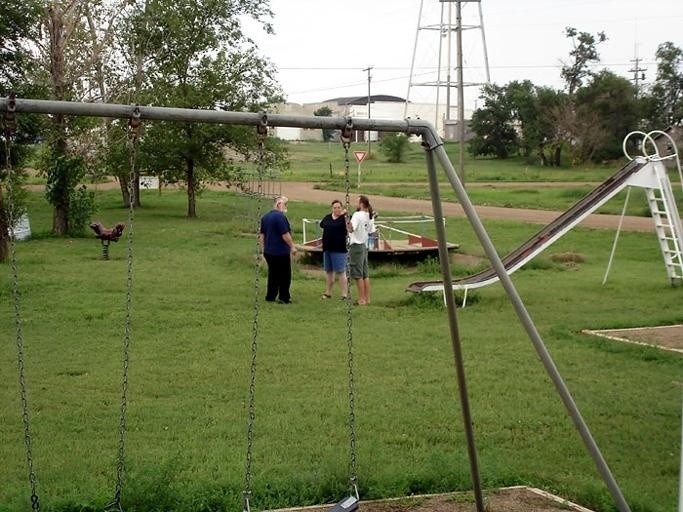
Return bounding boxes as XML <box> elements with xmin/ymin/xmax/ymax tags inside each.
<box><xmin>243</xmin><ymin>113</ymin><xmax>360</xmax><ymax>512</ymax></box>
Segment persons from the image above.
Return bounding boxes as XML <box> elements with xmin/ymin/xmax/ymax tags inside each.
<box><xmin>343</xmin><ymin>195</ymin><xmax>376</xmax><ymax>306</ymax></box>
<box><xmin>318</xmin><ymin>199</ymin><xmax>353</xmax><ymax>301</ymax></box>
<box><xmin>257</xmin><ymin>195</ymin><xmax>297</xmax><ymax>304</ymax></box>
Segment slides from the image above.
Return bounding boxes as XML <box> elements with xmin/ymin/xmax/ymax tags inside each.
<box><xmin>406</xmin><ymin>156</ymin><xmax>646</xmax><ymax>294</ymax></box>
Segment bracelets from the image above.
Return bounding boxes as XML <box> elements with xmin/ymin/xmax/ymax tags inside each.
<box><xmin>344</xmin><ymin>215</ymin><xmax>350</xmax><ymax>225</ymax></box>
